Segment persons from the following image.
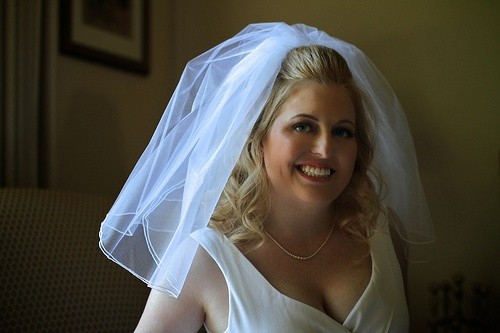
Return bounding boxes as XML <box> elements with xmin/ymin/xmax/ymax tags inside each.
<box><xmin>98</xmin><ymin>20</ymin><xmax>433</xmax><ymax>332</ymax></box>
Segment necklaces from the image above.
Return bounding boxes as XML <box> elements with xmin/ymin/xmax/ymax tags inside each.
<box><xmin>262</xmin><ymin>206</ymin><xmax>339</xmax><ymax>259</ymax></box>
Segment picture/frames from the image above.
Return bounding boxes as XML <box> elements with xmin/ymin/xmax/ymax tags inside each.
<box><xmin>57</xmin><ymin>0</ymin><xmax>151</xmax><ymax>77</ymax></box>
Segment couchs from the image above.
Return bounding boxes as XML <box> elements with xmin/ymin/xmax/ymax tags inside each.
<box><xmin>1</xmin><ymin>189</ymin><xmax>151</xmax><ymax>333</ymax></box>
